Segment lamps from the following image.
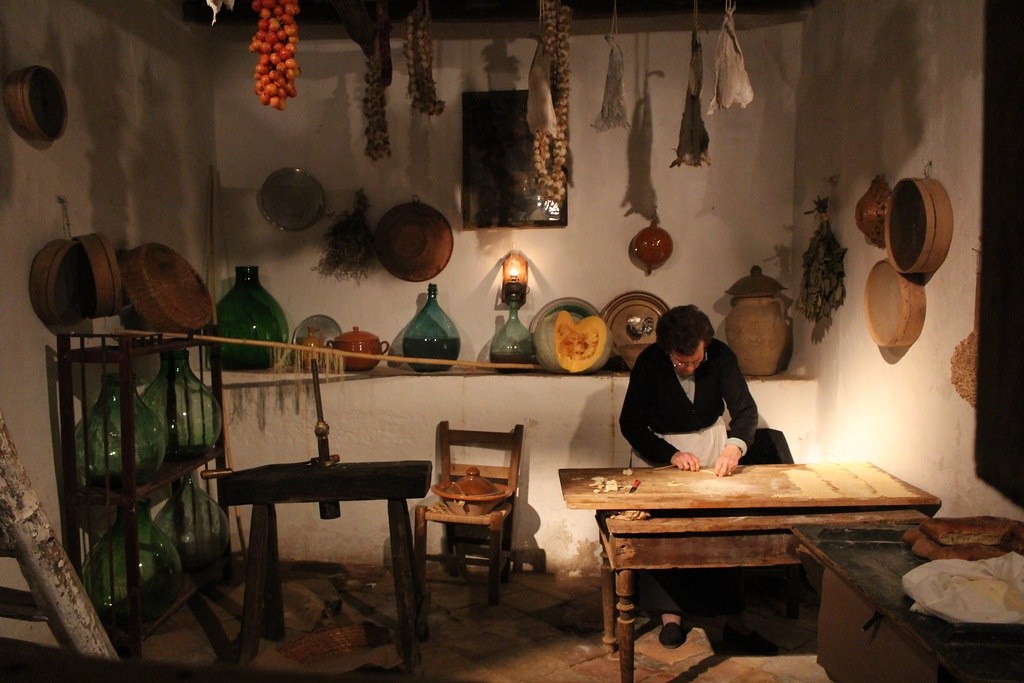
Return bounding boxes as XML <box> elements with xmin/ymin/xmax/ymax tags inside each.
<box><xmin>489</xmin><ymin>251</ymin><xmax>533</xmax><ymax>373</ymax></box>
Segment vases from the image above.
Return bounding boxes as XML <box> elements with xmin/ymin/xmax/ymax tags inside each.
<box><xmin>154</xmin><ymin>473</ymin><xmax>229</xmax><ymax>572</ymax></box>
<box><xmin>82</xmin><ymin>498</ymin><xmax>182</xmax><ymax>625</ymax></box>
<box><xmin>74</xmin><ymin>373</ymin><xmax>166</xmax><ymax>486</ymax></box>
<box><xmin>402</xmin><ymin>284</ymin><xmax>461</xmax><ymax>372</ymax></box>
<box><xmin>139</xmin><ymin>348</ymin><xmax>223</xmax><ymax>457</ymax></box>
<box><xmin>205</xmin><ymin>266</ymin><xmax>289</xmax><ymax>369</ymax></box>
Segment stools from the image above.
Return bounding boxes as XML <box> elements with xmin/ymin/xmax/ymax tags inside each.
<box><xmin>219</xmin><ymin>460</ymin><xmax>433</xmax><ymax>673</ymax></box>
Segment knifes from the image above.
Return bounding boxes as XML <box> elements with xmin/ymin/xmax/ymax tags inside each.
<box><xmin>629</xmin><ymin>480</ymin><xmax>641</xmax><ymax>493</ymax></box>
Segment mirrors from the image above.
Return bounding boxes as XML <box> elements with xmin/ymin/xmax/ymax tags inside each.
<box><xmin>460</xmin><ymin>88</ymin><xmax>568</xmax><ymax>230</ymax></box>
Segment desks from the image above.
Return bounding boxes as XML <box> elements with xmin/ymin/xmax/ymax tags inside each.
<box><xmin>789</xmin><ymin>516</ymin><xmax>1024</xmax><ymax>683</ymax></box>
<box><xmin>598</xmin><ymin>507</ymin><xmax>942</xmax><ymax>683</ymax></box>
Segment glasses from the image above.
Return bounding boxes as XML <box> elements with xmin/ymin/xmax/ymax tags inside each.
<box><xmin>668</xmin><ymin>346</ymin><xmax>708</xmax><ymax>368</ymax></box>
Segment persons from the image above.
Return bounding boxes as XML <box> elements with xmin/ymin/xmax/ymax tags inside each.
<box><xmin>620</xmin><ymin>305</ymin><xmax>758</xmax><ymax>650</ymax></box>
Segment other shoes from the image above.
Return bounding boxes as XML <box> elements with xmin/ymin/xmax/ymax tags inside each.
<box><xmin>659</xmin><ymin>615</ymin><xmax>681</xmax><ymax>649</ymax></box>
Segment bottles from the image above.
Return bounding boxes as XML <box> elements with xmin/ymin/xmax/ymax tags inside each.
<box><xmin>402</xmin><ymin>284</ymin><xmax>460</xmax><ymax>372</ymax></box>
<box><xmin>211</xmin><ymin>266</ymin><xmax>289</xmax><ymax>370</ymax></box>
<box><xmin>302</xmin><ymin>325</ymin><xmax>323</xmax><ymax>373</ymax></box>
<box><xmin>75</xmin><ymin>348</ymin><xmax>231</xmax><ymax>628</ymax></box>
<box><xmin>491</xmin><ymin>292</ymin><xmax>536</xmax><ymax>374</ymax></box>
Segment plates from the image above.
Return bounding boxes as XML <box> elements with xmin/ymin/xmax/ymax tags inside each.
<box><xmin>529</xmin><ymin>291</ymin><xmax>671</xmax><ymax>374</ymax></box>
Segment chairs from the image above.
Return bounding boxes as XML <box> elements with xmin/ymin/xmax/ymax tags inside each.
<box><xmin>414</xmin><ymin>421</ymin><xmax>524</xmax><ymax>606</ymax></box>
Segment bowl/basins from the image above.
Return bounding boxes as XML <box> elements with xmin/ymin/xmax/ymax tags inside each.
<box><xmin>326</xmin><ymin>326</ymin><xmax>389</xmax><ymax>373</ymax></box>
<box><xmin>432</xmin><ymin>467</ymin><xmax>515</xmax><ymax>517</ymax></box>
<box><xmin>620</xmin><ymin>343</ymin><xmax>653</xmax><ymax>372</ymax></box>
<box><xmin>630</xmin><ymin>220</ymin><xmax>672</xmax><ymax>274</ymax></box>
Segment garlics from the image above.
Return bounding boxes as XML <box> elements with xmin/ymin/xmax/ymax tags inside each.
<box><xmin>530</xmin><ymin>0</ymin><xmax>573</xmax><ymax>203</ymax></box>
<box><xmin>363</xmin><ymin>12</ymin><xmax>444</xmax><ymax>163</ymax></box>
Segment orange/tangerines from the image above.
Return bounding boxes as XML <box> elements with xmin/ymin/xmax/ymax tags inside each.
<box><xmin>247</xmin><ymin>0</ymin><xmax>301</xmax><ymax>112</ymax></box>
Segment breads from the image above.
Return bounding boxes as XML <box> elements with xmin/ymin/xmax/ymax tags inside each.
<box><xmin>903</xmin><ymin>515</ymin><xmax>1024</xmax><ymax>563</ymax></box>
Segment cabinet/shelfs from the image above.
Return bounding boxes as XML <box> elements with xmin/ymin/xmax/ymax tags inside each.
<box><xmin>56</xmin><ymin>330</ymin><xmax>233</xmax><ymax>659</ymax></box>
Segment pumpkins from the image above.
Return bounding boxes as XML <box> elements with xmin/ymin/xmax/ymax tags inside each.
<box><xmin>535</xmin><ymin>309</ymin><xmax>613</xmax><ymax>374</ymax></box>
